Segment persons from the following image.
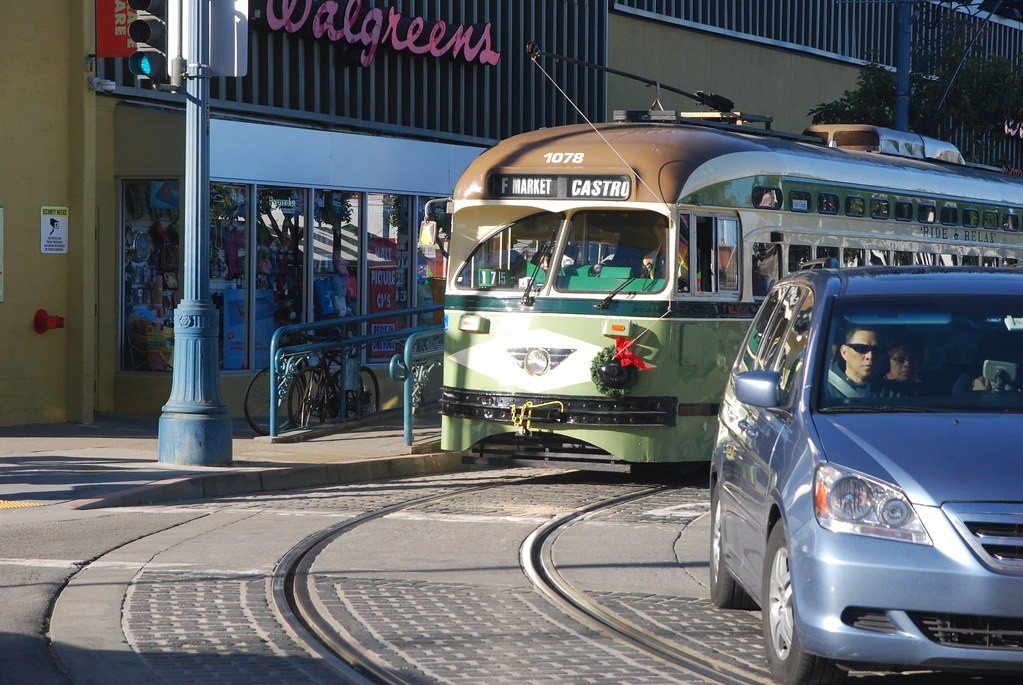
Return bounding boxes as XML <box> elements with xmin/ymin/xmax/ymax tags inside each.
<box><xmin>530</xmin><ymin>250</ymin><xmax>552</xmax><ymax>271</ymax></box>
<box><xmin>824</xmin><ymin>327</ymin><xmax>941</xmax><ymax>399</ymax></box>
<box><xmin>640</xmin><ymin>211</ymin><xmax>702</xmax><ymax>294</ymax></box>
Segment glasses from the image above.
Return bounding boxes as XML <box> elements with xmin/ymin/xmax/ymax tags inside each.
<box><xmin>655</xmin><ymin>223</ymin><xmax>669</xmax><ymax>230</ymax></box>
<box><xmin>846</xmin><ymin>344</ymin><xmax>883</xmax><ymax>355</ymax></box>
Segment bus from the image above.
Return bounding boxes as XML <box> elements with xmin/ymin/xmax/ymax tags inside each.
<box><xmin>416</xmin><ymin>1</ymin><xmax>1022</xmax><ymax>465</ymax></box>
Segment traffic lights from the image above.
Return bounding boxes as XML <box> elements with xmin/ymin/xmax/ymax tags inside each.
<box><xmin>126</xmin><ymin>1</ymin><xmax>169</xmax><ymax>85</ymax></box>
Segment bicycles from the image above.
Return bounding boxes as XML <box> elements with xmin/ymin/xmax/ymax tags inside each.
<box><xmin>242</xmin><ymin>325</ymin><xmax>380</xmax><ymax>436</ymax></box>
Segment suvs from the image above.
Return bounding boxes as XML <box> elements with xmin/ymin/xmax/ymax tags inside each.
<box><xmin>710</xmin><ymin>258</ymin><xmax>1022</xmax><ymax>684</ymax></box>
